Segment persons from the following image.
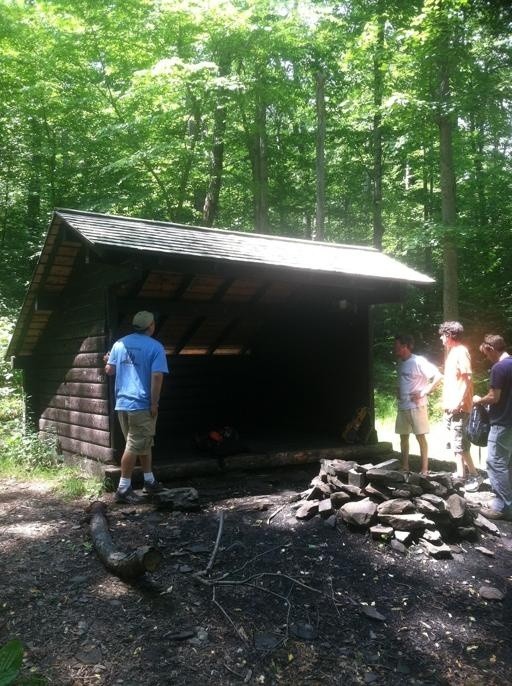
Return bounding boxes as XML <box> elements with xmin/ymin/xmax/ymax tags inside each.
<box><xmin>434</xmin><ymin>320</ymin><xmax>483</xmax><ymax>491</ymax></box>
<box><xmin>466</xmin><ymin>333</ymin><xmax>511</xmax><ymax>519</ymax></box>
<box><xmin>103</xmin><ymin>311</ymin><xmax>172</xmax><ymax>504</ymax></box>
<box><xmin>392</xmin><ymin>334</ymin><xmax>440</xmax><ymax>478</ymax></box>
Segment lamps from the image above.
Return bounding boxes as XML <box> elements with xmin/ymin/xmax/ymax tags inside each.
<box><xmin>85</xmin><ymin>246</ymin><xmax>89</xmax><ymax>264</ymax></box>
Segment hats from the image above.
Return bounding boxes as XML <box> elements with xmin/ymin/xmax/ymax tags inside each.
<box><xmin>132</xmin><ymin>311</ymin><xmax>154</xmax><ymax>330</ymax></box>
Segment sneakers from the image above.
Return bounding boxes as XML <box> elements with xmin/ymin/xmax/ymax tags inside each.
<box><xmin>485</xmin><ymin>497</ymin><xmax>504</xmax><ymax>512</ymax></box>
<box><xmin>142</xmin><ymin>480</ymin><xmax>170</xmax><ymax>496</ymax></box>
<box><xmin>464</xmin><ymin>474</ymin><xmax>484</xmax><ymax>492</ymax></box>
<box><xmin>113</xmin><ymin>485</ymin><xmax>147</xmax><ymax>504</ymax></box>
<box><xmin>452</xmin><ymin>472</ymin><xmax>469</xmax><ymax>481</ymax></box>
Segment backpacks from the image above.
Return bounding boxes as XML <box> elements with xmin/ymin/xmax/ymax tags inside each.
<box><xmin>459</xmin><ymin>402</ymin><xmax>491</xmax><ymax>448</ymax></box>
